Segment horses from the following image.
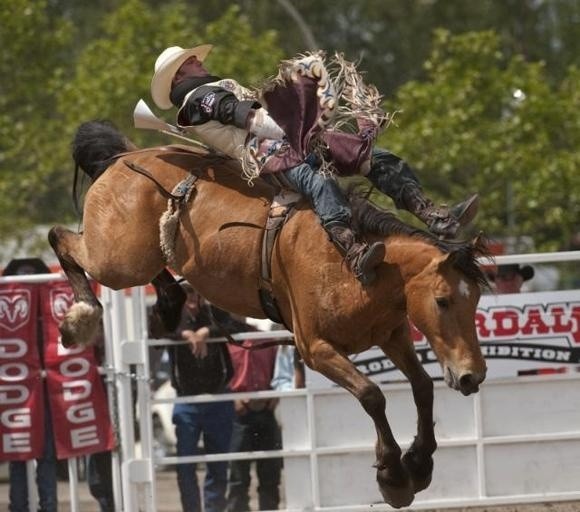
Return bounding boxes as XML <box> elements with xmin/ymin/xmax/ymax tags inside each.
<box><xmin>47</xmin><ymin>117</ymin><xmax>495</xmax><ymax>510</ymax></box>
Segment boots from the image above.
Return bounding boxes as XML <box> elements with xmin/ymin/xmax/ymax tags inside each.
<box><xmin>326</xmin><ymin>220</ymin><xmax>386</xmax><ymax>287</ymax></box>
<box><xmin>404</xmin><ymin>185</ymin><xmax>479</xmax><ymax>240</ymax></box>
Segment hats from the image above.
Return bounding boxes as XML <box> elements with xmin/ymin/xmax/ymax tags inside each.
<box><xmin>151</xmin><ymin>43</ymin><xmax>214</xmax><ymax>111</ymax></box>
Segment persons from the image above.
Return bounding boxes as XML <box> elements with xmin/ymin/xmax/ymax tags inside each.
<box><xmin>132</xmin><ymin>41</ymin><xmax>478</xmax><ymax>282</ymax></box>
<box><xmin>493</xmin><ymin>261</ymin><xmax>535</xmax><ymax>294</ymax></box>
<box><xmin>224</xmin><ymin>325</ymin><xmax>284</xmax><ymax>511</ymax></box>
<box><xmin>150</xmin><ymin>278</ymin><xmax>258</xmax><ymax>512</ymax></box>
<box><xmin>79</xmin><ymin>345</ymin><xmax>139</xmax><ymax>512</ymax></box>
<box><xmin>2</xmin><ymin>254</ymin><xmax>59</xmax><ymax>512</ymax></box>
